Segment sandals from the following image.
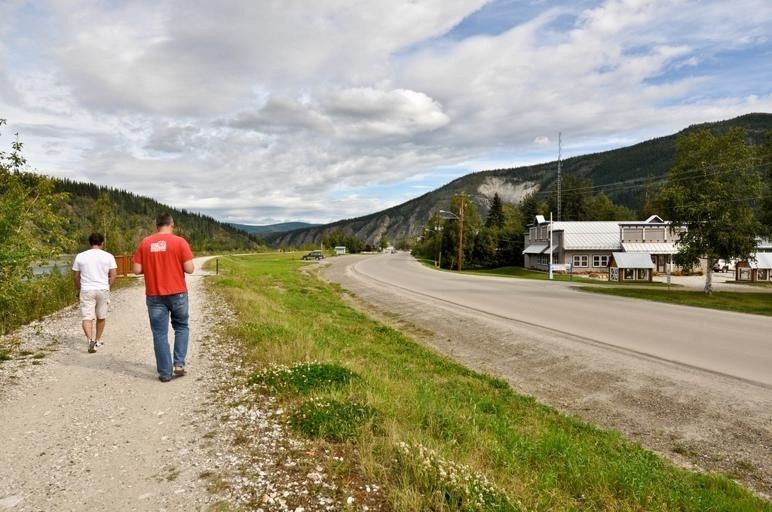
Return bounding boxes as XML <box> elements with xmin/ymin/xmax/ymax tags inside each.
<box><xmin>174</xmin><ymin>366</ymin><xmax>187</xmax><ymax>376</ymax></box>
<box><xmin>158</xmin><ymin>375</ymin><xmax>170</xmax><ymax>382</ymax></box>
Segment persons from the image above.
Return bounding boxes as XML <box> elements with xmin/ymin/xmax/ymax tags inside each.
<box><xmin>73</xmin><ymin>236</ymin><xmax>118</xmax><ymax>352</ymax></box>
<box><xmin>131</xmin><ymin>213</ymin><xmax>195</xmax><ymax>382</ymax></box>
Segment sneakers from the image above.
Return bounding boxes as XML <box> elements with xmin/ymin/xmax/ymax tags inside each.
<box><xmin>88</xmin><ymin>339</ymin><xmax>103</xmax><ymax>353</ymax></box>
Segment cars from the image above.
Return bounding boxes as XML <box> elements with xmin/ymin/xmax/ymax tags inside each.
<box><xmin>304</xmin><ymin>251</ymin><xmax>325</xmax><ymax>260</ymax></box>
<box><xmin>713</xmin><ymin>258</ymin><xmax>741</xmax><ymax>273</ymax></box>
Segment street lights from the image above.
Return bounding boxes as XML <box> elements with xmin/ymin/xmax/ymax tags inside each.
<box><xmin>425</xmin><ymin>210</ymin><xmax>463</xmax><ymax>271</ymax></box>
